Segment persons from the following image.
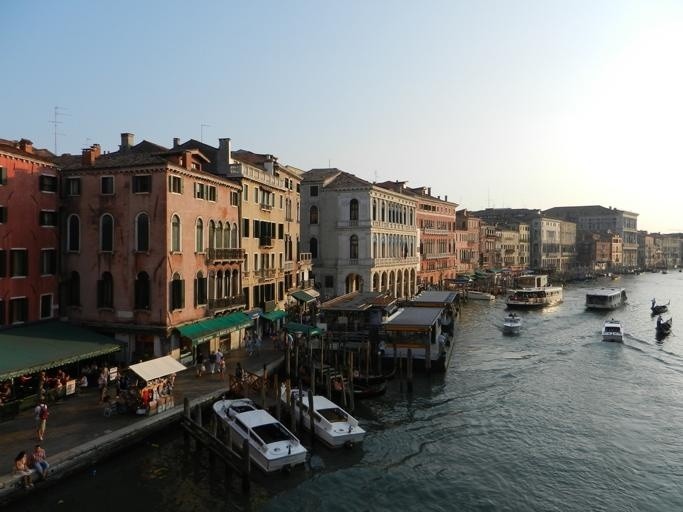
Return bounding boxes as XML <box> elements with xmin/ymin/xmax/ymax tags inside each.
<box><xmin>1</xmin><ymin>370</ymin><xmax>87</xmax><ymax>401</ymax></box>
<box><xmin>97</xmin><ymin>367</ymin><xmax>121</xmax><ymax>403</ymax></box>
<box><xmin>29</xmin><ymin>445</ymin><xmax>49</xmax><ymax>480</ymax></box>
<box><xmin>192</xmin><ymin>349</ymin><xmax>226</xmax><ymax>377</ymax></box>
<box><xmin>235</xmin><ymin>363</ymin><xmax>245</xmax><ymax>385</ymax></box>
<box><xmin>242</xmin><ymin>330</ymin><xmax>264</xmax><ymax>358</ymax></box>
<box><xmin>15</xmin><ymin>450</ymin><xmax>34</xmax><ymax>489</ymax></box>
<box><xmin>33</xmin><ymin>399</ymin><xmax>48</xmax><ymax>440</ymax></box>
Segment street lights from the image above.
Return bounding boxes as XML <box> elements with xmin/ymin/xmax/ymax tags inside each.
<box><xmin>286</xmin><ymin>293</ymin><xmax>292</xmax><ymax>323</ymax></box>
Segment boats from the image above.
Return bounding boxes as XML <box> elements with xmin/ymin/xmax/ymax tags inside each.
<box><xmin>612</xmin><ymin>275</ymin><xmax>621</xmax><ymax>281</ymax></box>
<box><xmin>503</xmin><ymin>316</ymin><xmax>523</xmax><ymax>336</ymax></box>
<box><xmin>586</xmin><ymin>287</ymin><xmax>627</xmax><ymax>312</ymax></box>
<box><xmin>460</xmin><ymin>291</ymin><xmax>495</xmax><ymax>302</ymax></box>
<box><xmin>505</xmin><ymin>287</ymin><xmax>564</xmax><ymax>311</ymax></box>
<box><xmin>602</xmin><ymin>319</ymin><xmax>625</xmax><ymax>344</ymax></box>
<box><xmin>651</xmin><ymin>299</ymin><xmax>671</xmax><ymax>315</ymax></box>
<box><xmin>376</xmin><ymin>290</ymin><xmax>461</xmax><ymax>372</ymax></box>
<box><xmin>655</xmin><ymin>318</ymin><xmax>672</xmax><ymax>337</ymax></box>
<box><xmin>210</xmin><ymin>348</ymin><xmax>377</xmax><ymax>475</ymax></box>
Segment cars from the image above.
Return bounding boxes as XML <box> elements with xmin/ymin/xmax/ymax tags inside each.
<box><xmin>465</xmin><ymin>279</ymin><xmax>473</xmax><ymax>287</ymax></box>
